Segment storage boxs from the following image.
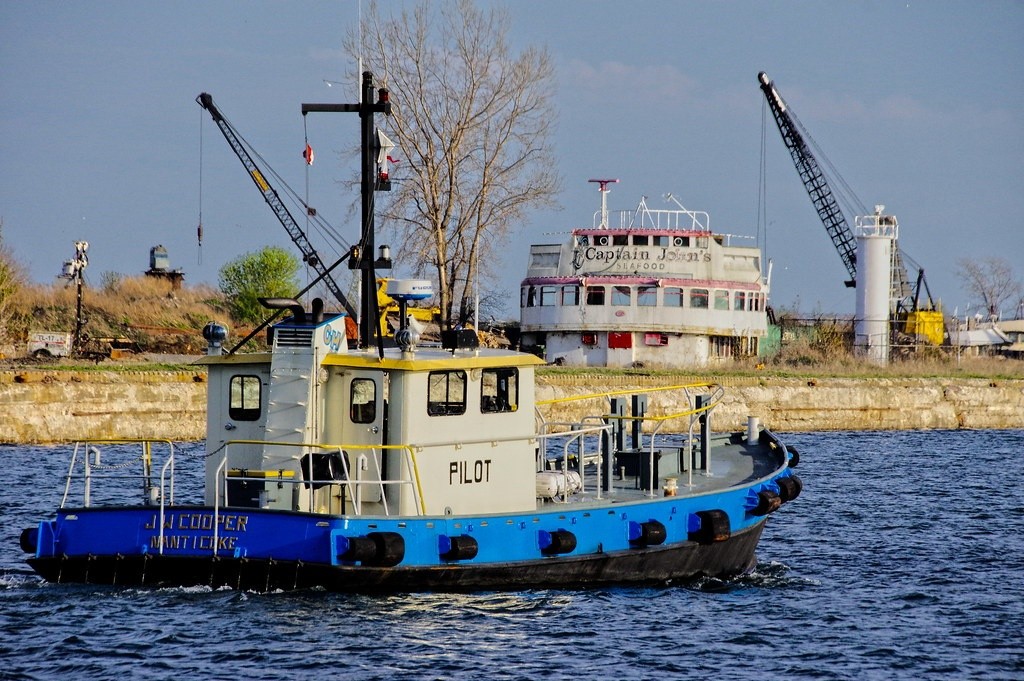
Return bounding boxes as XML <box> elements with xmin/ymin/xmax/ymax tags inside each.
<box><xmin>222</xmin><ymin>469</ymin><xmax>294</xmax><ymax>510</ymax></box>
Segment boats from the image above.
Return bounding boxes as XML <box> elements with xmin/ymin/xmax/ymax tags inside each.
<box><xmin>22</xmin><ymin>70</ymin><xmax>802</xmax><ymax>594</ymax></box>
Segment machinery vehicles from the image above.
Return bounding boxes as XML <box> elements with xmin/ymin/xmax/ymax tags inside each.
<box><xmin>194</xmin><ymin>93</ymin><xmax>442</xmax><ymax>348</ymax></box>
<box><xmin>757</xmin><ymin>70</ymin><xmax>951</xmax><ymax>359</ymax></box>
<box><xmin>25</xmin><ymin>330</ymin><xmax>135</xmax><ymax>363</ymax></box>
